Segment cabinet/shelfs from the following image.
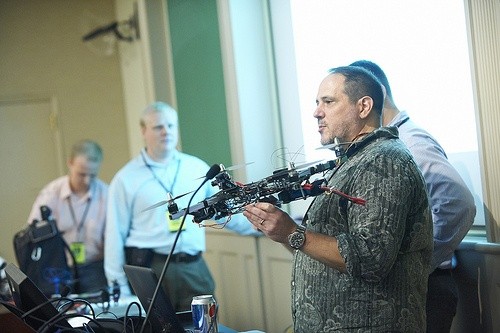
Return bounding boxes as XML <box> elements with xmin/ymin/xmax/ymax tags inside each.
<box><xmin>203</xmin><ymin>235</ymin><xmax>500</xmax><ymax>333</ymax></box>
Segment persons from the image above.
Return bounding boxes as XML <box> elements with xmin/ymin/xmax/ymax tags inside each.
<box><xmin>242</xmin><ymin>66</ymin><xmax>433</xmax><ymax>333</ymax></box>
<box><xmin>103</xmin><ymin>100</ymin><xmax>265</xmax><ymax>320</ymax></box>
<box><xmin>24</xmin><ymin>137</ymin><xmax>109</xmax><ymax>293</ymax></box>
<box><xmin>349</xmin><ymin>60</ymin><xmax>477</xmax><ymax>333</ymax></box>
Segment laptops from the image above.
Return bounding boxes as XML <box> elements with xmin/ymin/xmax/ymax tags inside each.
<box><xmin>122</xmin><ymin>265</ymin><xmax>190</xmax><ymax>333</ymax></box>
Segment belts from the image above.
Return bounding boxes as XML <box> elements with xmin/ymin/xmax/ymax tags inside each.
<box><xmin>154</xmin><ymin>251</ymin><xmax>202</xmax><ymax>263</ymax></box>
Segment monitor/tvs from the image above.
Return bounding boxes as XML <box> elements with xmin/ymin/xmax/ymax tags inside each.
<box><xmin>4</xmin><ymin>263</ymin><xmax>73</xmax><ymax>333</ymax></box>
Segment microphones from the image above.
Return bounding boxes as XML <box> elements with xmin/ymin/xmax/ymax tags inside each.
<box><xmin>138</xmin><ymin>164</ymin><xmax>221</xmax><ymax>333</ymax></box>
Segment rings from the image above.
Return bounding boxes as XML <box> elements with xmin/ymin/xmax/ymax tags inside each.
<box><xmin>260</xmin><ymin>219</ymin><xmax>265</xmax><ymax>224</ymax></box>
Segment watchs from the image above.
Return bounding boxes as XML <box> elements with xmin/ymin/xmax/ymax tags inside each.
<box><xmin>288</xmin><ymin>225</ymin><xmax>306</xmax><ymax>250</ymax></box>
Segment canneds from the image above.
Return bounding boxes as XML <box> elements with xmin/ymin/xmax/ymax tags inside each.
<box><xmin>191</xmin><ymin>295</ymin><xmax>218</xmax><ymax>333</ymax></box>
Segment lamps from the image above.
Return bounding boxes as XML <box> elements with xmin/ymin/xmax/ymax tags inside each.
<box><xmin>81</xmin><ymin>0</ymin><xmax>141</xmax><ymax>59</ymax></box>
